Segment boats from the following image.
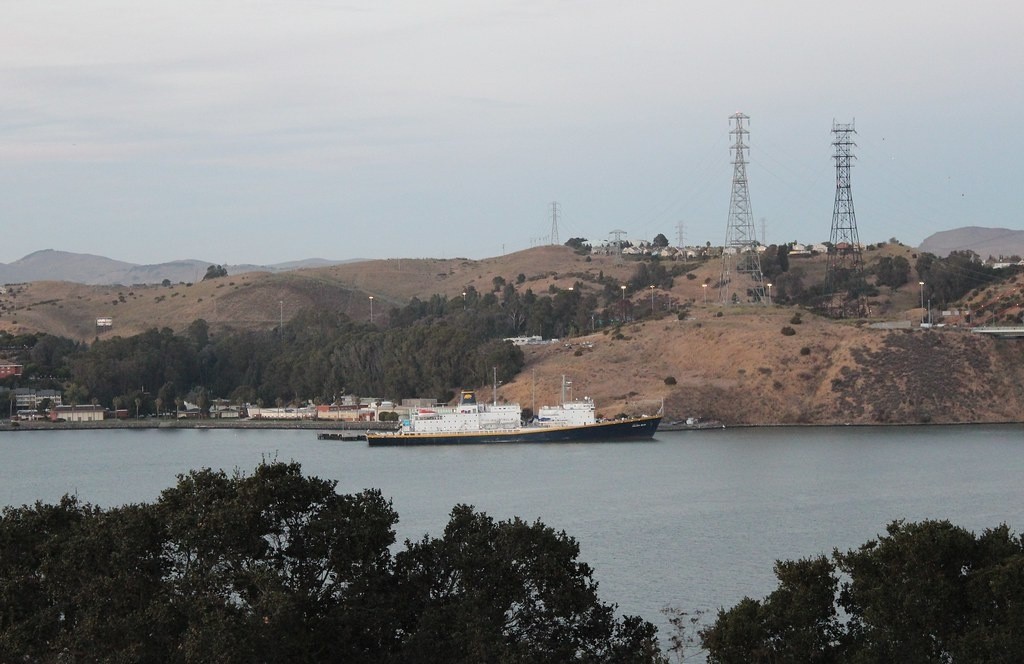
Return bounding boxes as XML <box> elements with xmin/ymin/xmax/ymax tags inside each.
<box><xmin>364</xmin><ymin>393</ymin><xmax>665</xmax><ymax>447</ymax></box>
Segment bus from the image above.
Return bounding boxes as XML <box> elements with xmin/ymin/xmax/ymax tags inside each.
<box><xmin>17</xmin><ymin>408</ymin><xmax>50</xmax><ymax>419</ymax></box>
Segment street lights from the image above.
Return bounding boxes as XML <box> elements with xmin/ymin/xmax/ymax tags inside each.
<box><xmin>917</xmin><ymin>280</ymin><xmax>926</xmax><ymax>323</ymax></box>
<box><xmin>566</xmin><ymin>382</ymin><xmax>573</xmax><ymax>402</ymax></box>
<box><xmin>280</xmin><ymin>301</ymin><xmax>283</xmax><ymax>327</ymax></box>
<box><xmin>532</xmin><ymin>369</ymin><xmax>535</xmax><ymax>416</ymax></box>
<box><xmin>462</xmin><ymin>292</ymin><xmax>466</xmax><ymax>311</ymax></box>
<box><xmin>650</xmin><ymin>285</ymin><xmax>655</xmax><ymax>310</ymax></box>
<box><xmin>562</xmin><ymin>374</ymin><xmax>565</xmax><ymax>403</ymax></box>
<box><xmin>621</xmin><ymin>285</ymin><xmax>626</xmax><ymax>321</ymax></box>
<box><xmin>766</xmin><ymin>283</ymin><xmax>772</xmax><ymax>302</ymax></box>
<box><xmin>369</xmin><ymin>296</ymin><xmax>373</xmax><ymax>323</ymax></box>
<box><xmin>493</xmin><ymin>366</ymin><xmax>498</xmax><ymax>405</ymax></box>
<box><xmin>702</xmin><ymin>285</ymin><xmax>708</xmax><ymax>304</ymax></box>
